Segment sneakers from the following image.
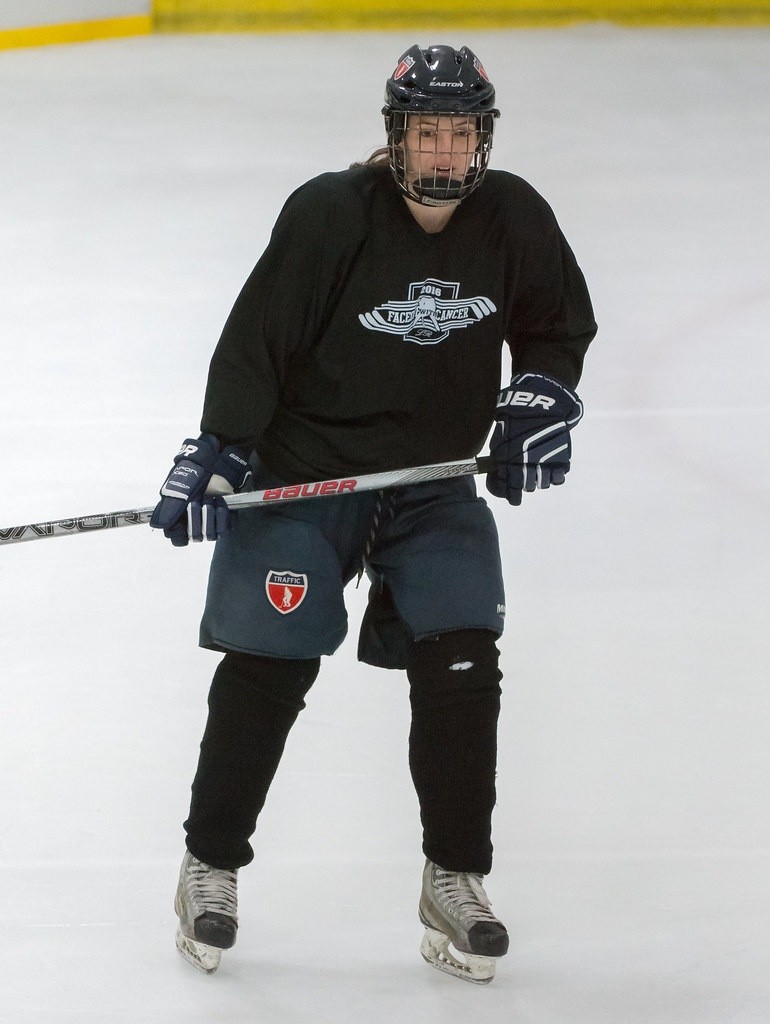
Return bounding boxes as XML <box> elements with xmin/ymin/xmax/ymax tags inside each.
<box><xmin>173</xmin><ymin>850</ymin><xmax>239</xmax><ymax>974</ymax></box>
<box><xmin>418</xmin><ymin>859</ymin><xmax>509</xmax><ymax>984</ymax></box>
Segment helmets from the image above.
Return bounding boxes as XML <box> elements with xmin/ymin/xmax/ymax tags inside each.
<box><xmin>381</xmin><ymin>46</ymin><xmax>500</xmax><ymax>208</ymax></box>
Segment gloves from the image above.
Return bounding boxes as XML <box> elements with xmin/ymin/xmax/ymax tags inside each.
<box><xmin>149</xmin><ymin>435</ymin><xmax>253</xmax><ymax>548</ymax></box>
<box><xmin>487</xmin><ymin>373</ymin><xmax>582</xmax><ymax>506</ymax></box>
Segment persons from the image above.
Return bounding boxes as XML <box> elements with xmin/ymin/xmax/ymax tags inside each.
<box><xmin>149</xmin><ymin>39</ymin><xmax>599</xmax><ymax>989</ymax></box>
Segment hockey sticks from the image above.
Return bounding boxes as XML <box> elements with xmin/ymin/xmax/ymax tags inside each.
<box><xmin>2</xmin><ymin>454</ymin><xmax>503</xmax><ymax>548</ymax></box>
